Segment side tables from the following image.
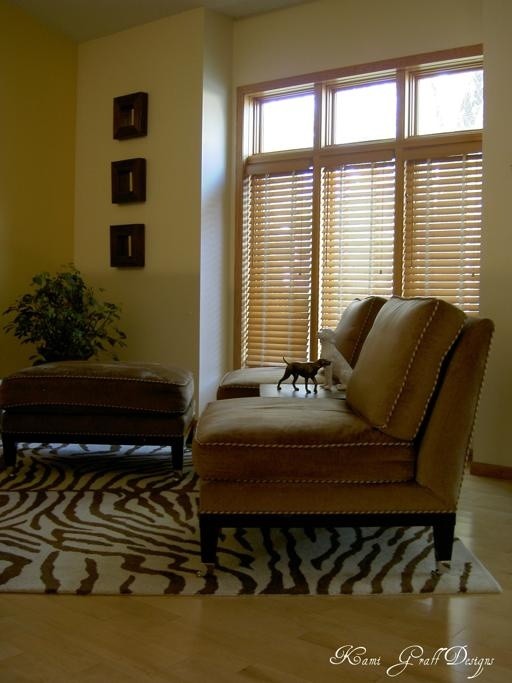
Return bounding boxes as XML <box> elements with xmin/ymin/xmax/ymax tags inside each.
<box><xmin>258</xmin><ymin>380</ymin><xmax>347</xmax><ymax>398</ymax></box>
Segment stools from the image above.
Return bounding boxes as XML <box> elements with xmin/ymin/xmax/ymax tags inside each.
<box><xmin>0</xmin><ymin>364</ymin><xmax>196</xmax><ymax>470</ymax></box>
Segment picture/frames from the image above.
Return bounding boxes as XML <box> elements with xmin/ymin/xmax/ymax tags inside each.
<box><xmin>110</xmin><ymin>223</ymin><xmax>146</xmax><ymax>269</ymax></box>
<box><xmin>111</xmin><ymin>157</ymin><xmax>146</xmax><ymax>204</ymax></box>
<box><xmin>113</xmin><ymin>92</ymin><xmax>148</xmax><ymax>140</ymax></box>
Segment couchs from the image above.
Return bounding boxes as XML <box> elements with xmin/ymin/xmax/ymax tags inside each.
<box><xmin>191</xmin><ymin>295</ymin><xmax>495</xmax><ymax>564</ymax></box>
<box><xmin>217</xmin><ymin>297</ymin><xmax>387</xmax><ymax>401</ymax></box>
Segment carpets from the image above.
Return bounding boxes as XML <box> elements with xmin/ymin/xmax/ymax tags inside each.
<box><xmin>0</xmin><ymin>437</ymin><xmax>502</xmax><ymax>597</ymax></box>
